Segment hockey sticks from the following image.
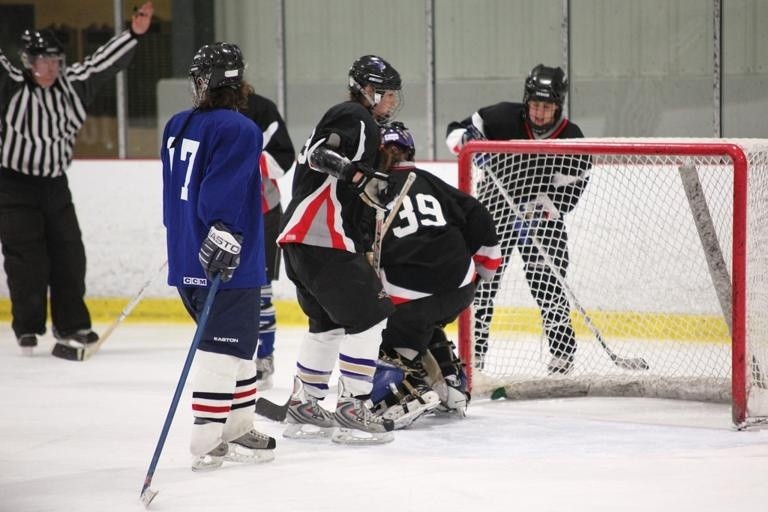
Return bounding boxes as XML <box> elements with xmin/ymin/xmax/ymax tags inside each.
<box><xmin>50</xmin><ymin>258</ymin><xmax>168</xmax><ymax>361</ymax></box>
<box><xmin>256</xmin><ymin>394</ymin><xmax>291</xmax><ymax>422</ymax></box>
<box><xmin>485</xmin><ymin>165</ymin><xmax>649</xmax><ymax>370</ymax></box>
<box><xmin>139</xmin><ymin>276</ymin><xmax>224</xmax><ymax>506</ymax></box>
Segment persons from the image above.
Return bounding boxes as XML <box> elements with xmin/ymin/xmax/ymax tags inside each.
<box><xmin>447</xmin><ymin>63</ymin><xmax>592</xmax><ymax>375</ymax></box>
<box><xmin>0</xmin><ymin>0</ymin><xmax>153</xmax><ymax>347</ymax></box>
<box><xmin>275</xmin><ymin>55</ymin><xmax>404</xmax><ymax>432</ymax></box>
<box><xmin>160</xmin><ymin>42</ymin><xmax>276</xmax><ymax>458</ymax></box>
<box><xmin>357</xmin><ymin>122</ymin><xmax>502</xmax><ymax>431</ymax></box>
<box><xmin>236</xmin><ymin>92</ymin><xmax>295</xmax><ymax>382</ymax></box>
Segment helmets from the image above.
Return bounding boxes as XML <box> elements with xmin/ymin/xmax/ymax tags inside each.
<box><xmin>348</xmin><ymin>55</ymin><xmax>403</xmax><ymax>124</ymax></box>
<box><xmin>378</xmin><ymin>121</ymin><xmax>415</xmax><ymax>160</ymax></box>
<box><xmin>188</xmin><ymin>41</ymin><xmax>245</xmax><ymax>110</ymax></box>
<box><xmin>19</xmin><ymin>28</ymin><xmax>66</xmax><ymax>77</ymax></box>
<box><xmin>520</xmin><ymin>64</ymin><xmax>569</xmax><ymax>133</ymax></box>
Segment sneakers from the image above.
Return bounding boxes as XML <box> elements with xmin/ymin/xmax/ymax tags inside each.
<box><xmin>53</xmin><ymin>329</ymin><xmax>99</xmax><ymax>343</ymax></box>
<box><xmin>17</xmin><ymin>333</ymin><xmax>37</xmax><ymax>346</ymax></box>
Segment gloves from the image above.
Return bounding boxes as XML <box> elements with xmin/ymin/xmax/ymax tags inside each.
<box><xmin>198</xmin><ymin>220</ymin><xmax>244</xmax><ymax>283</ymax></box>
<box><xmin>354</xmin><ymin>170</ymin><xmax>390</xmax><ymax>212</ymax></box>
